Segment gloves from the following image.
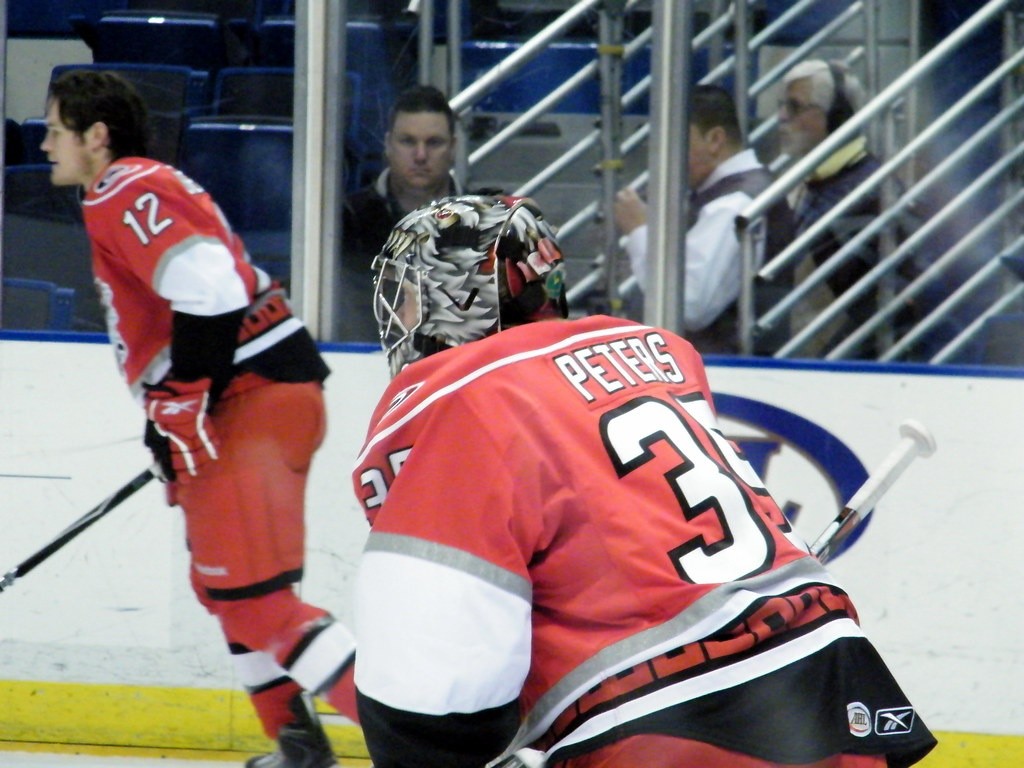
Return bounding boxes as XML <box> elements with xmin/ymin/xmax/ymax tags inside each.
<box><xmin>143</xmin><ymin>377</ymin><xmax>221</xmax><ymax>485</ymax></box>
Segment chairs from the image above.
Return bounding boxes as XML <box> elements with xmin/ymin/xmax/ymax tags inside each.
<box><xmin>0</xmin><ymin>0</ymin><xmax>851</xmax><ymax>331</ymax></box>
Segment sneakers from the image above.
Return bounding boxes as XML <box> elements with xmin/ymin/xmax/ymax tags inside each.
<box><xmin>244</xmin><ymin>689</ymin><xmax>337</xmax><ymax>768</ymax></box>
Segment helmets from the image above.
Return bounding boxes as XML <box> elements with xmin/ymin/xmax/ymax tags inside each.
<box><xmin>369</xmin><ymin>194</ymin><xmax>565</xmax><ymax>381</ymax></box>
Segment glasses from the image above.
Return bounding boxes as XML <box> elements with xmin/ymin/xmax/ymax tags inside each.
<box><xmin>776</xmin><ymin>98</ymin><xmax>826</xmax><ymax>116</ymax></box>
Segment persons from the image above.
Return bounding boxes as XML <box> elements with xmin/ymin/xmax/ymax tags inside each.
<box><xmin>38</xmin><ymin>67</ymin><xmax>366</xmax><ymax>767</ymax></box>
<box><xmin>777</xmin><ymin>58</ymin><xmax>991</xmax><ymax>364</ymax></box>
<box><xmin>352</xmin><ymin>192</ymin><xmax>939</xmax><ymax>768</ymax></box>
<box><xmin>340</xmin><ymin>83</ymin><xmax>469</xmax><ymax>345</ymax></box>
<box><xmin>612</xmin><ymin>85</ymin><xmax>797</xmax><ymax>360</ymax></box>
